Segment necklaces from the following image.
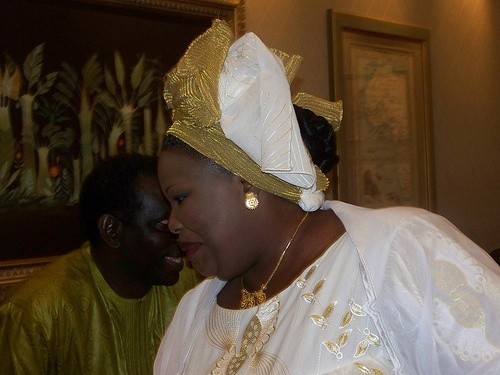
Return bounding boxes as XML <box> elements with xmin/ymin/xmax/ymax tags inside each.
<box><xmin>241</xmin><ymin>211</ymin><xmax>310</xmax><ymax>310</ymax></box>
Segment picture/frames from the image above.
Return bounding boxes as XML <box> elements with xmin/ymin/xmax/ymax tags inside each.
<box><xmin>0</xmin><ymin>0</ymin><xmax>247</xmax><ymax>288</ymax></box>
<box><xmin>326</xmin><ymin>7</ymin><xmax>438</xmax><ymax>216</ymax></box>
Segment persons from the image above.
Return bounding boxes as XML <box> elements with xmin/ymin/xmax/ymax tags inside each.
<box><xmin>0</xmin><ymin>152</ymin><xmax>200</xmax><ymax>375</ymax></box>
<box><xmin>152</xmin><ymin>18</ymin><xmax>500</xmax><ymax>375</ymax></box>
